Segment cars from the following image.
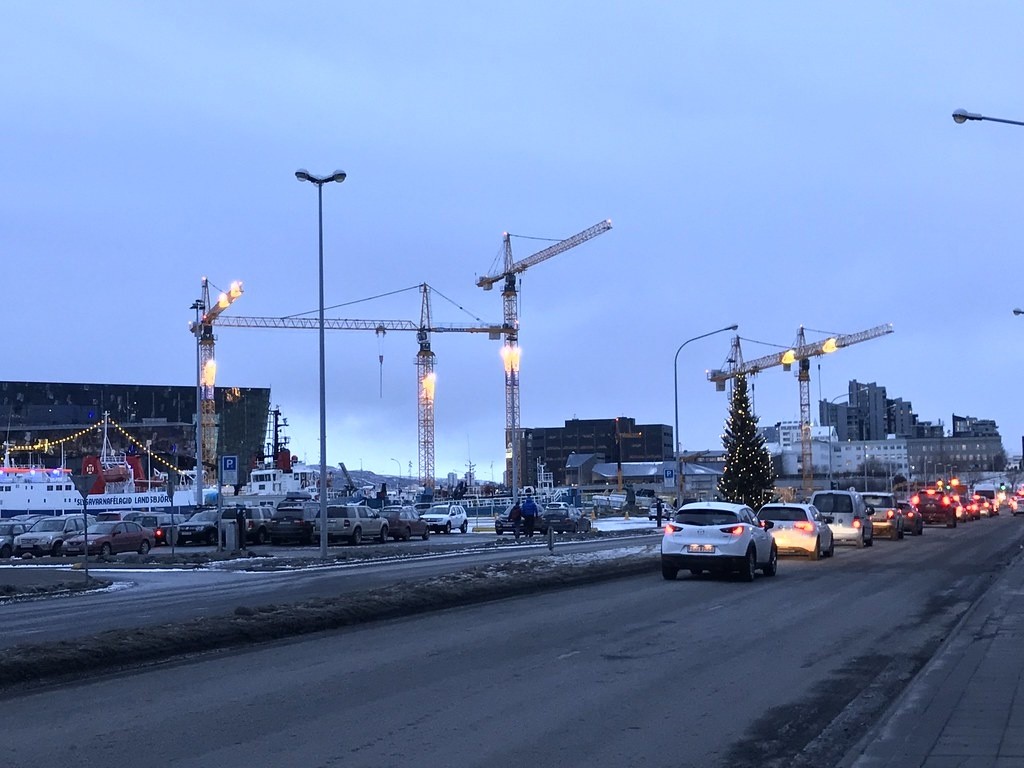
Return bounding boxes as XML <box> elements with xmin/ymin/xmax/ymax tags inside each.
<box><xmin>897</xmin><ymin>503</ymin><xmax>923</xmax><ymax>536</ymax></box>
<box><xmin>913</xmin><ymin>484</ymin><xmax>1024</xmax><ymax>528</ymax></box>
<box><xmin>648</xmin><ymin>503</ymin><xmax>670</xmax><ymax>521</ymax></box>
<box><xmin>495</xmin><ymin>501</ymin><xmax>592</xmax><ymax>534</ymax></box>
<box><xmin>60</xmin><ymin>521</ymin><xmax>156</xmax><ymax>558</ymax></box>
<box><xmin>756</xmin><ymin>503</ymin><xmax>835</xmax><ymax>560</ymax></box>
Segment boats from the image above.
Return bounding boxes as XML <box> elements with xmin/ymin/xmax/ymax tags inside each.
<box><xmin>0</xmin><ymin>410</ymin><xmax>235</xmax><ymax>515</ymax></box>
<box><xmin>222</xmin><ymin>410</ymin><xmax>330</xmax><ymax>507</ymax></box>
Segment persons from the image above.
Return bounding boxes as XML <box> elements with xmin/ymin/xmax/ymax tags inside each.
<box><xmin>508</xmin><ymin>502</ymin><xmax>522</xmax><ymax>538</ymax></box>
<box><xmin>520</xmin><ymin>496</ymin><xmax>538</xmax><ymax>538</ymax></box>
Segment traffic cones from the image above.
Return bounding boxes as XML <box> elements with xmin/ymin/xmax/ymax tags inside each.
<box><xmin>591</xmin><ymin>510</ymin><xmax>596</xmax><ymax>520</ymax></box>
<box><xmin>624</xmin><ymin>512</ymin><xmax>630</xmax><ymax>520</ymax></box>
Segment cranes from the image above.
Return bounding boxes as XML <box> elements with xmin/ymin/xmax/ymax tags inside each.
<box><xmin>476</xmin><ymin>220</ymin><xmax>614</xmax><ymax>487</ymax></box>
<box><xmin>211</xmin><ymin>281</ymin><xmax>502</xmax><ymax>486</ymax></box>
<box><xmin>705</xmin><ymin>324</ymin><xmax>895</xmax><ymax>493</ymax></box>
<box><xmin>188</xmin><ymin>278</ymin><xmax>243</xmax><ymax>488</ymax></box>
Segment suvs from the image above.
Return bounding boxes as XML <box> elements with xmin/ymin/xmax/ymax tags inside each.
<box><xmin>860</xmin><ymin>492</ymin><xmax>905</xmax><ymax>540</ymax></box>
<box><xmin>661</xmin><ymin>502</ymin><xmax>778</xmax><ymax>582</ymax></box>
<box><xmin>1</xmin><ymin>493</ymin><xmax>470</xmax><ymax>557</ymax></box>
<box><xmin>809</xmin><ymin>490</ymin><xmax>875</xmax><ymax>548</ymax></box>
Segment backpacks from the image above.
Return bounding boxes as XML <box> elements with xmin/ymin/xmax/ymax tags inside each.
<box><xmin>512</xmin><ymin>508</ymin><xmax>520</xmax><ymax>519</ymax></box>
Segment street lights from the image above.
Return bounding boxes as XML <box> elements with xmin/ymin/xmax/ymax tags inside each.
<box><xmin>675</xmin><ymin>325</ymin><xmax>738</xmax><ymax>510</ymax></box>
<box><xmin>295</xmin><ymin>169</ymin><xmax>346</xmax><ymax>561</ymax></box>
<box><xmin>360</xmin><ymin>458</ymin><xmax>362</xmax><ymax>476</ymax></box>
<box><xmin>828</xmin><ymin>387</ymin><xmax>869</xmax><ymax>490</ymax></box>
<box><xmin>391</xmin><ymin>459</ymin><xmax>401</xmax><ymax>482</ymax></box>
